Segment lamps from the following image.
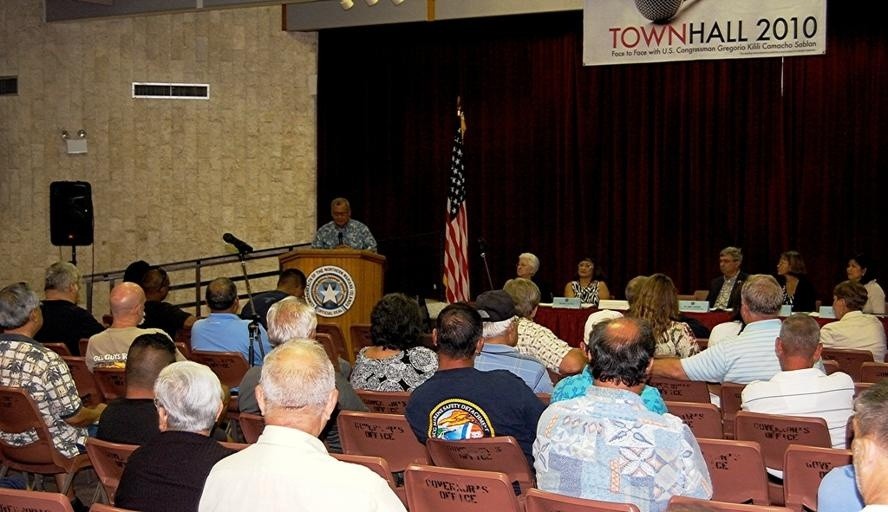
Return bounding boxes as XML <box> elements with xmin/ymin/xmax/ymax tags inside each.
<box><xmin>60</xmin><ymin>128</ymin><xmax>89</xmax><ymax>154</ymax></box>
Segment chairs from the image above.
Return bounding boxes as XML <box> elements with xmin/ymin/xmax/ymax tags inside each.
<box><xmin>0</xmin><ymin>324</ymin><xmax>888</xmax><ymax>512</ymax></box>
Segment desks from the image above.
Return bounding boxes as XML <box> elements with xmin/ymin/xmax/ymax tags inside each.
<box><xmin>533</xmin><ymin>307</ymin><xmax>887</xmax><ymax>346</ymax></box>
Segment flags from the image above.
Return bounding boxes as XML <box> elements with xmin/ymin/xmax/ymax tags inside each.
<box><xmin>443</xmin><ymin>108</ymin><xmax>471</xmax><ymax>306</ymax></box>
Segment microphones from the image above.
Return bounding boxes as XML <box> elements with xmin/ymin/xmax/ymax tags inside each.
<box><xmin>338</xmin><ymin>232</ymin><xmax>344</xmax><ymax>244</ymax></box>
<box><xmin>477</xmin><ymin>237</ymin><xmax>489</xmax><ymax>256</ymax></box>
<box><xmin>223</xmin><ymin>233</ymin><xmax>254</xmax><ymax>252</ymax></box>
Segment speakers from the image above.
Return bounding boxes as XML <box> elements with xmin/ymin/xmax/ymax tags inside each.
<box><xmin>50</xmin><ymin>180</ymin><xmax>93</xmax><ymax>246</ymax></box>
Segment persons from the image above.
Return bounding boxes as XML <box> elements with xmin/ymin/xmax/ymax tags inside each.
<box><xmin>1</xmin><ymin>248</ymin><xmax>887</xmax><ymax>511</ymax></box>
<box><xmin>312</xmin><ymin>197</ymin><xmax>378</xmax><ymax>252</ymax></box>
